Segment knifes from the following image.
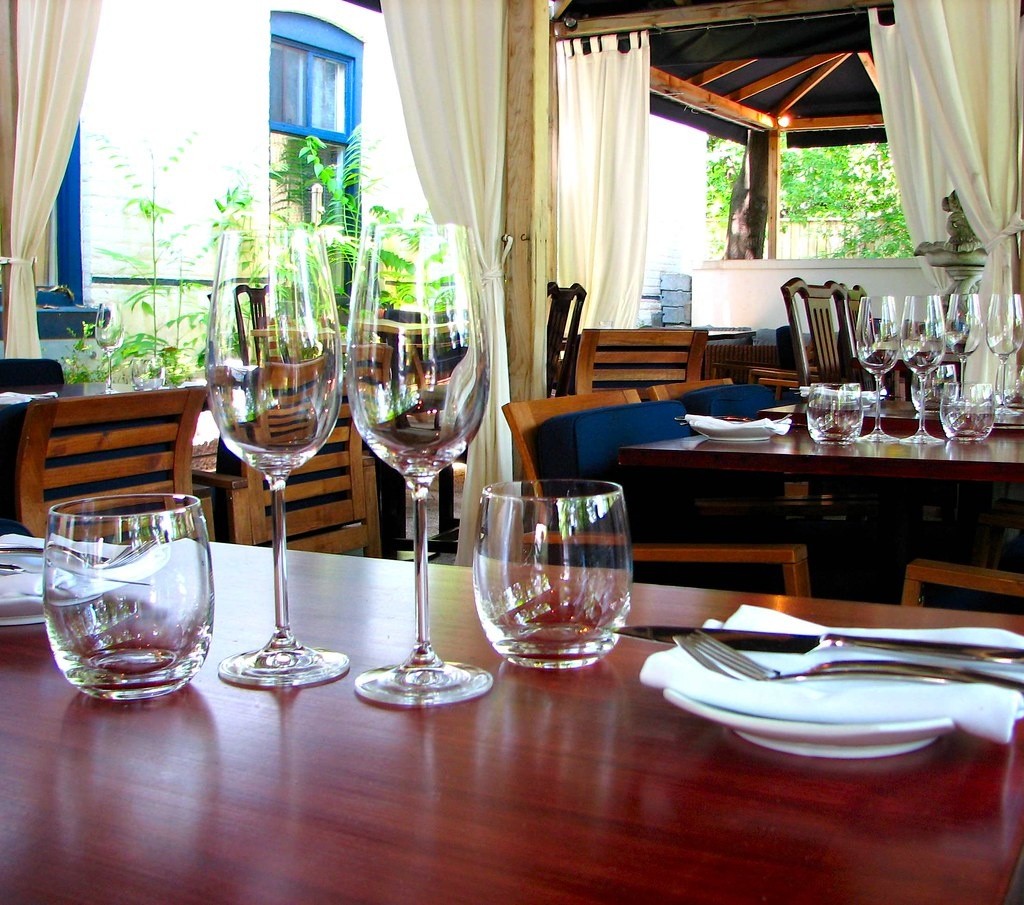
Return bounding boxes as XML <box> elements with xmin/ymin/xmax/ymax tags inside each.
<box><xmin>0</xmin><ymin>561</ymin><xmax>153</xmax><ymax>587</ymax></box>
<box><xmin>674</xmin><ymin>416</ymin><xmax>807</xmax><ymax>429</ymax></box>
<box><xmin>611</xmin><ymin>626</ymin><xmax>1023</xmax><ymax>665</ymax></box>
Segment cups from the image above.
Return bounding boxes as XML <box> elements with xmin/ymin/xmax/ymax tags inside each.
<box><xmin>471</xmin><ymin>479</ymin><xmax>634</xmax><ymax>668</ymax></box>
<box><xmin>806</xmin><ymin>383</ymin><xmax>864</xmax><ymax>445</ymax></box>
<box><xmin>132</xmin><ymin>357</ymin><xmax>166</xmax><ymax>391</ymax></box>
<box><xmin>44</xmin><ymin>494</ymin><xmax>213</xmax><ymax>700</ymax></box>
<box><xmin>910</xmin><ymin>364</ymin><xmax>1024</xmax><ymax>413</ymax></box>
<box><xmin>941</xmin><ymin>382</ymin><xmax>995</xmax><ymax>442</ymax></box>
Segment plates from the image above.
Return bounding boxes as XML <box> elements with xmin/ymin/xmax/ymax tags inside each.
<box><xmin>861</xmin><ymin>398</ymin><xmax>877</xmax><ymax>406</ymax></box>
<box><xmin>0</xmin><ymin>554</ymin><xmax>170</xmax><ymax>626</ymax></box>
<box><xmin>663</xmin><ymin>688</ymin><xmax>956</xmax><ymax>760</ymax></box>
<box><xmin>690</xmin><ymin>425</ymin><xmax>776</xmax><ymax>442</ymax></box>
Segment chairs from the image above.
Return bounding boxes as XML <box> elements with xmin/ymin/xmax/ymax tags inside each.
<box><xmin>19</xmin><ymin>281</ymin><xmax>1024</xmax><ymax>618</ymax></box>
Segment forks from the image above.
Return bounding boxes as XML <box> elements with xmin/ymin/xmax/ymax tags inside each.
<box><xmin>686</xmin><ymin>624</ymin><xmax>1023</xmax><ymax>679</ymax></box>
<box><xmin>0</xmin><ymin>540</ymin><xmax>155</xmax><ymax>570</ymax></box>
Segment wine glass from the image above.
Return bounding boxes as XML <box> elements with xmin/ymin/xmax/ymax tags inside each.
<box><xmin>95</xmin><ymin>303</ymin><xmax>125</xmax><ymax>395</ymax></box>
<box><xmin>944</xmin><ymin>294</ymin><xmax>984</xmax><ymax>408</ymax></box>
<box><xmin>984</xmin><ymin>293</ymin><xmax>1021</xmax><ymax>416</ymax></box>
<box><xmin>206</xmin><ymin>231</ymin><xmax>349</xmax><ymax>691</ymax></box>
<box><xmin>348</xmin><ymin>224</ymin><xmax>494</xmax><ymax>709</ymax></box>
<box><xmin>900</xmin><ymin>295</ymin><xmax>944</xmax><ymax>444</ymax></box>
<box><xmin>855</xmin><ymin>296</ymin><xmax>902</xmax><ymax>443</ymax></box>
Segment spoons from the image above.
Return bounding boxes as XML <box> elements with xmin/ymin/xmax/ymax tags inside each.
<box><xmin>672</xmin><ymin>633</ymin><xmax>1024</xmax><ymax>697</ymax></box>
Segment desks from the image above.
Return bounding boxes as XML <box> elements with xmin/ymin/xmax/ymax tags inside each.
<box><xmin>0</xmin><ymin>382</ymin><xmax>209</xmax><ymax>436</ymax></box>
<box><xmin>619</xmin><ymin>430</ymin><xmax>1024</xmax><ymax>610</ymax></box>
<box><xmin>758</xmin><ymin>399</ymin><xmax>1024</xmax><ymax>436</ymax></box>
<box><xmin>0</xmin><ymin>535</ymin><xmax>1024</xmax><ymax>905</ymax></box>
<box><xmin>706</xmin><ymin>330</ymin><xmax>759</xmax><ymax>346</ymax></box>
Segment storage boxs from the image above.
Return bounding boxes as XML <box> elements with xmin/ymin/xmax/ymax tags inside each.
<box><xmin>638</xmin><ymin>270</ymin><xmax>691</xmax><ymax>328</ymax></box>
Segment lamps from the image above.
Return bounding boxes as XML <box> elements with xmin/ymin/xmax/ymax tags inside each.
<box><xmin>563</xmin><ymin>9</ymin><xmax>577</xmax><ymax>28</ymax></box>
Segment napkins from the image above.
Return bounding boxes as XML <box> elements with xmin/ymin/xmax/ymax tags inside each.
<box><xmin>800</xmin><ymin>386</ymin><xmax>887</xmax><ymax>403</ymax></box>
<box><xmin>638</xmin><ymin>604</ymin><xmax>1024</xmax><ymax>744</ymax></box>
<box><xmin>0</xmin><ymin>533</ymin><xmax>171</xmax><ymax>616</ymax></box>
<box><xmin>0</xmin><ymin>391</ymin><xmax>58</xmax><ymax>405</ymax></box>
<box><xmin>685</xmin><ymin>414</ymin><xmax>791</xmax><ymax>435</ymax></box>
